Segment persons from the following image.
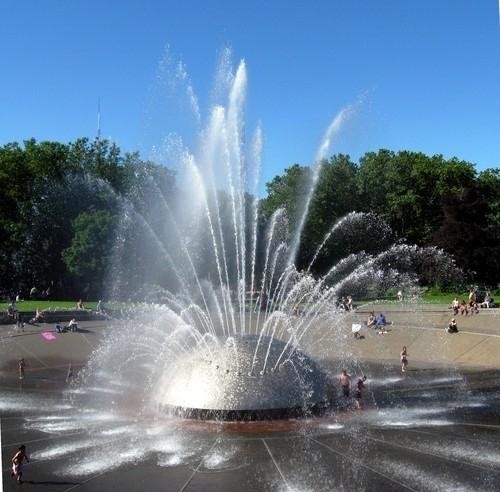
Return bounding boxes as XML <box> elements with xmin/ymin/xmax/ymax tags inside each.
<box><xmin>335</xmin><ymin>368</ymin><xmax>351</xmax><ymax>398</ymax></box>
<box><xmin>399</xmin><ymin>345</ymin><xmax>408</xmax><ymax>373</ymax></box>
<box><xmin>1</xmin><ymin>279</ymin><xmax>110</xmax><ymax>336</ymax></box>
<box><xmin>352</xmin><ymin>375</ymin><xmax>367</xmax><ymax>410</ymax></box>
<box><xmin>9</xmin><ymin>444</ymin><xmax>29</xmax><ymax>485</ymax></box>
<box><xmin>325</xmin><ymin>286</ymin><xmax>497</xmax><ymax>340</ymax></box>
